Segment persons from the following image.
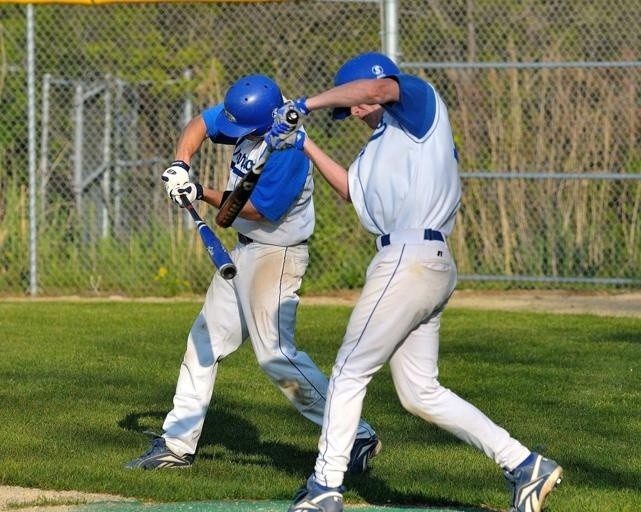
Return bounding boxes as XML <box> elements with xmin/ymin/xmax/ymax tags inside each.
<box><xmin>126</xmin><ymin>76</ymin><xmax>382</xmax><ymax>473</ymax></box>
<box><xmin>264</xmin><ymin>53</ymin><xmax>563</xmax><ymax>512</ymax></box>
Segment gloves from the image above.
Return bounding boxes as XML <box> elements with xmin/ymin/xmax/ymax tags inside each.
<box><xmin>161</xmin><ymin>160</ymin><xmax>203</xmax><ymax>208</ymax></box>
<box><xmin>265</xmin><ymin>96</ymin><xmax>310</xmax><ymax>151</ymax></box>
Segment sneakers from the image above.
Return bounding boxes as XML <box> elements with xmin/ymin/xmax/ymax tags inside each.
<box><xmin>348</xmin><ymin>434</ymin><xmax>382</xmax><ymax>473</ymax></box>
<box><xmin>504</xmin><ymin>452</ymin><xmax>564</xmax><ymax>512</ymax></box>
<box><xmin>125</xmin><ymin>438</ymin><xmax>193</xmax><ymax>470</ymax></box>
<box><xmin>288</xmin><ymin>473</ymin><xmax>345</xmax><ymax>512</ymax></box>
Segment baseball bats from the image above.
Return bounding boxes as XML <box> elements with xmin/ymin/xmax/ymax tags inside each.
<box><xmin>180</xmin><ymin>194</ymin><xmax>236</xmax><ymax>278</ymax></box>
<box><xmin>215</xmin><ymin>110</ymin><xmax>298</xmax><ymax>227</ymax></box>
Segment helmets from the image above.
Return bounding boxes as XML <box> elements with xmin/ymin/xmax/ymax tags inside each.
<box><xmin>216</xmin><ymin>75</ymin><xmax>284</xmax><ymax>138</ymax></box>
<box><xmin>333</xmin><ymin>52</ymin><xmax>400</xmax><ymax>120</ymax></box>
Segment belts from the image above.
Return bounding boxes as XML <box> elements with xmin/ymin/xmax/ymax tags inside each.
<box><xmin>238</xmin><ymin>232</ymin><xmax>308</xmax><ymax>245</ymax></box>
<box><xmin>381</xmin><ymin>229</ymin><xmax>444</xmax><ymax>246</ymax></box>
